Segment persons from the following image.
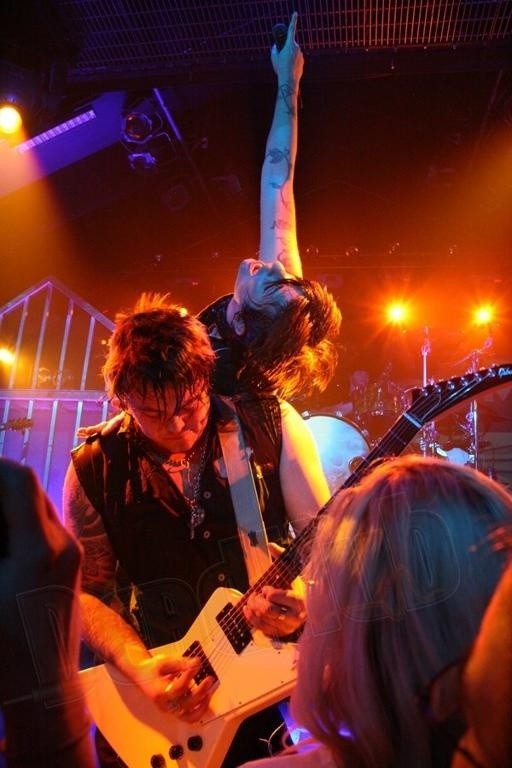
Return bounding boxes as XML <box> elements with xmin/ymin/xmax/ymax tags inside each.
<box><xmin>63</xmin><ymin>292</ymin><xmax>332</xmax><ymax>768</ymax></box>
<box><xmin>75</xmin><ymin>11</ymin><xmax>343</xmax><ymax>438</ymax></box>
<box><xmin>290</xmin><ymin>456</ymin><xmax>512</xmax><ymax>768</ymax></box>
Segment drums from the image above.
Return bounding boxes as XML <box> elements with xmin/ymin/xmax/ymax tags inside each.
<box><xmin>299</xmin><ymin>409</ymin><xmax>373</xmax><ymax>498</ymax></box>
<box><xmin>400</xmin><ymin>386</ymin><xmax>420</xmax><ymax>413</ymax></box>
<box><xmin>351</xmin><ymin>379</ymin><xmax>405</xmax><ymax>445</ymax></box>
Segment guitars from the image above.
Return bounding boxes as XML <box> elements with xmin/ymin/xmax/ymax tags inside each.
<box><xmin>77</xmin><ymin>362</ymin><xmax>512</xmax><ymax>767</ymax></box>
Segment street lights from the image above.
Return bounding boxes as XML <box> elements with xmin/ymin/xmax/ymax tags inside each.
<box><xmin>383</xmin><ymin>295</ymin><xmax>499</xmax><ymax>470</ymax></box>
